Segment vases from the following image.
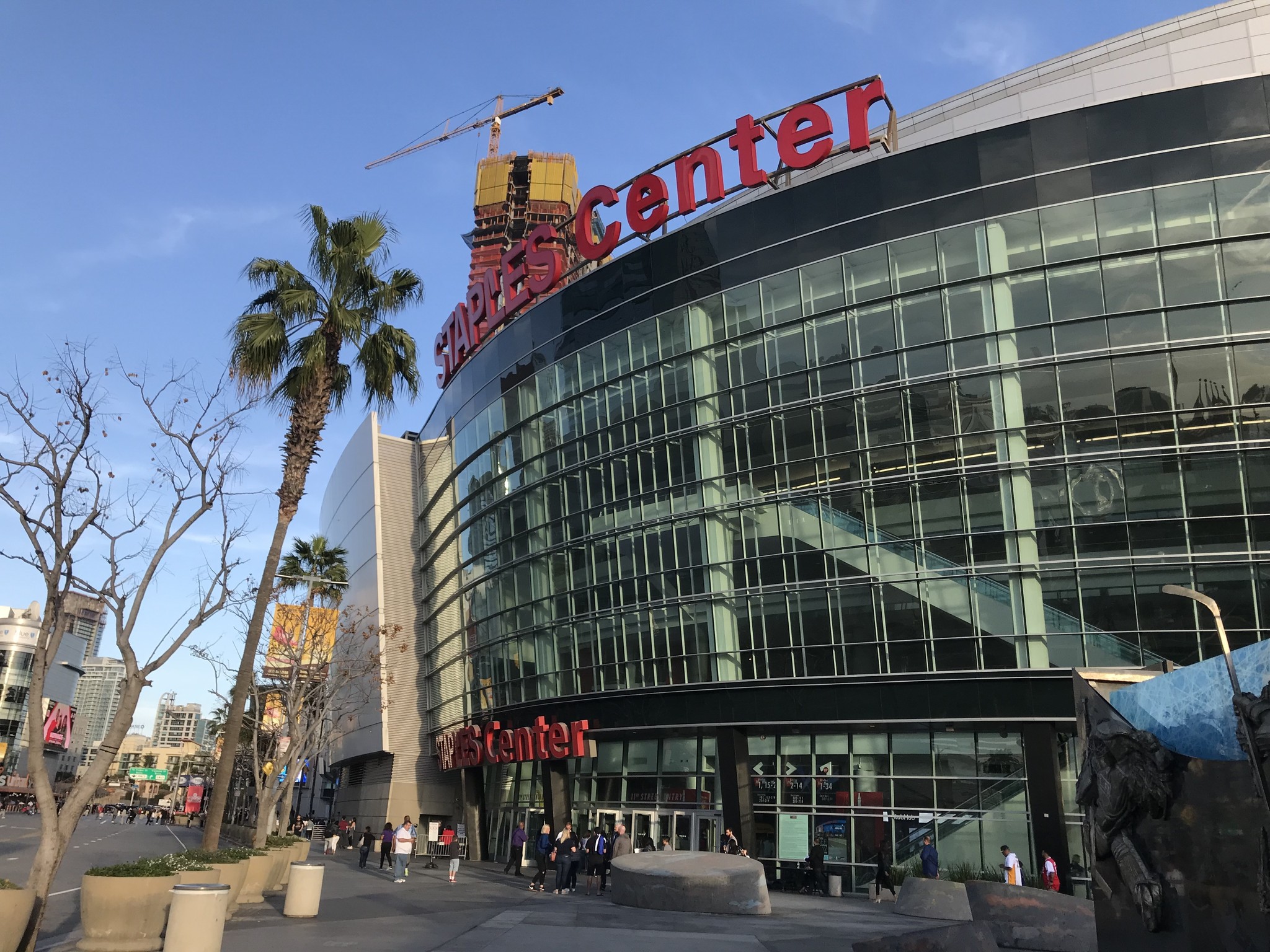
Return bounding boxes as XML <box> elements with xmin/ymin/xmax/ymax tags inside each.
<box><xmin>228</xmin><ymin>859</ymin><xmax>251</xmax><ymax>911</ymax></box>
<box><xmin>202</xmin><ymin>863</ymin><xmax>245</xmax><ymax>920</ymax></box>
<box><xmin>236</xmin><ymin>855</ymin><xmax>274</xmax><ymax>904</ymax></box>
<box><xmin>161</xmin><ymin>867</ymin><xmax>221</xmax><ymax>937</ymax></box>
<box><xmin>75</xmin><ymin>875</ymin><xmax>182</xmax><ymax>952</ymax></box>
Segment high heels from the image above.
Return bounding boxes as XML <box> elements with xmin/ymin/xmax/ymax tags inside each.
<box><xmin>873</xmin><ymin>895</ymin><xmax>881</xmax><ymax>903</ymax></box>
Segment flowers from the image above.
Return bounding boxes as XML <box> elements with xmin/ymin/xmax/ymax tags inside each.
<box><xmin>84</xmin><ymin>846</ymin><xmax>267</xmax><ymax>877</ymax></box>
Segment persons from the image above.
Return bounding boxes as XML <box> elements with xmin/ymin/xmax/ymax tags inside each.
<box><xmin>393</xmin><ymin>820</ymin><xmax>416</xmax><ymax>883</ymax></box>
<box><xmin>919</xmin><ymin>836</ymin><xmax>939</xmax><ymax>879</ymax></box>
<box><xmin>346</xmin><ymin>817</ymin><xmax>357</xmax><ymax>849</ymax></box>
<box><xmin>612</xmin><ymin>825</ymin><xmax>632</xmax><ymax>859</ymax></box>
<box><xmin>378</xmin><ymin>822</ymin><xmax>394</xmax><ymax>870</ymax></box>
<box><xmin>222</xmin><ymin>808</ymin><xmax>340</xmax><ymax>855</ymax></box>
<box><xmin>392</xmin><ymin>815</ymin><xmax>417</xmax><ymax>877</ymax></box>
<box><xmin>610</xmin><ymin>822</ymin><xmax>623</xmax><ymax>861</ymax></box>
<box><xmin>1042</xmin><ymin>849</ymin><xmax>1060</xmax><ymax>893</ymax></box>
<box><xmin>1070</xmin><ymin>854</ymin><xmax>1093</xmax><ymax>894</ymax></box>
<box><xmin>999</xmin><ymin>845</ymin><xmax>1025</xmax><ymax>886</ymax></box>
<box><xmin>0</xmin><ymin>798</ymin><xmax>207</xmax><ymax>829</ymax></box>
<box><xmin>338</xmin><ymin>816</ymin><xmax>348</xmax><ymax>849</ymax></box>
<box><xmin>723</xmin><ymin>827</ymin><xmax>750</xmax><ymax>858</ymax></box>
<box><xmin>661</xmin><ymin>836</ymin><xmax>672</xmax><ymax>851</ymax></box>
<box><xmin>504</xmin><ymin>822</ymin><xmax>529</xmax><ymax>876</ymax></box>
<box><xmin>358</xmin><ymin>826</ymin><xmax>375</xmax><ymax>869</ymax></box>
<box><xmin>873</xmin><ymin>840</ymin><xmax>898</xmax><ymax>904</ymax></box>
<box><xmin>640</xmin><ymin>837</ymin><xmax>656</xmax><ymax>852</ymax></box>
<box><xmin>799</xmin><ymin>839</ymin><xmax>831</xmax><ymax>897</ymax></box>
<box><xmin>528</xmin><ymin>824</ymin><xmax>552</xmax><ymax>892</ymax></box>
<box><xmin>439</xmin><ymin>825</ymin><xmax>455</xmax><ymax>860</ymax></box>
<box><xmin>449</xmin><ymin>835</ymin><xmax>461</xmax><ymax>882</ymax></box>
<box><xmin>45</xmin><ymin>709</ymin><xmax>68</xmax><ymax>748</ymax></box>
<box><xmin>554</xmin><ymin>822</ymin><xmax>611</xmax><ymax>896</ymax></box>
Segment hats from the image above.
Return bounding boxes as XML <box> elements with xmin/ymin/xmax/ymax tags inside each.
<box><xmin>1001</xmin><ymin>845</ymin><xmax>1009</xmax><ymax>851</ymax></box>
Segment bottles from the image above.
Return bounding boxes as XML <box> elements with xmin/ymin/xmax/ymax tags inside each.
<box><xmin>797</xmin><ymin>861</ymin><xmax>799</xmax><ymax>869</ymax></box>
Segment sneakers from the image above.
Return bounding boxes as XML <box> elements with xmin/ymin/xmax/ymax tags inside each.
<box><xmin>394</xmin><ymin>879</ymin><xmax>402</xmax><ymax>883</ymax></box>
<box><xmin>387</xmin><ymin>866</ymin><xmax>392</xmax><ymax>870</ymax></box>
<box><xmin>540</xmin><ymin>887</ymin><xmax>545</xmax><ymax>892</ymax></box>
<box><xmin>400</xmin><ymin>879</ymin><xmax>405</xmax><ymax>882</ymax></box>
<box><xmin>528</xmin><ymin>885</ymin><xmax>537</xmax><ymax>891</ymax></box>
<box><xmin>449</xmin><ymin>879</ymin><xmax>451</xmax><ymax>882</ymax></box>
<box><xmin>451</xmin><ymin>879</ymin><xmax>457</xmax><ymax>882</ymax></box>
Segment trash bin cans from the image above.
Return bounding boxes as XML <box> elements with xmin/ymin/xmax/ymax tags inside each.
<box><xmin>161</xmin><ymin>884</ymin><xmax>230</xmax><ymax>951</ymax></box>
<box><xmin>828</xmin><ymin>875</ymin><xmax>842</xmax><ymax>897</ymax></box>
<box><xmin>283</xmin><ymin>860</ymin><xmax>325</xmax><ymax>918</ymax></box>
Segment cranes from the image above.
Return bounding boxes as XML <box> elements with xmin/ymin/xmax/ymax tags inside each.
<box><xmin>364</xmin><ymin>84</ymin><xmax>565</xmax><ymax>170</ymax></box>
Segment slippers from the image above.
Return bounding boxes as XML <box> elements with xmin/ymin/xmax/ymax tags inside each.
<box><xmin>597</xmin><ymin>893</ymin><xmax>603</xmax><ymax>896</ymax></box>
<box><xmin>585</xmin><ymin>893</ymin><xmax>590</xmax><ymax>895</ymax></box>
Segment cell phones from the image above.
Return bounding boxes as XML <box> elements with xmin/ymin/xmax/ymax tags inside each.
<box><xmin>414</xmin><ymin>837</ymin><xmax>417</xmax><ymax>839</ymax></box>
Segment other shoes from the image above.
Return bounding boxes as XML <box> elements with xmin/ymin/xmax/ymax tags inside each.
<box><xmin>347</xmin><ymin>847</ymin><xmax>350</xmax><ymax>849</ymax></box>
<box><xmin>553</xmin><ymin>890</ymin><xmax>558</xmax><ymax>894</ymax></box>
<box><xmin>567</xmin><ymin>888</ymin><xmax>570</xmax><ymax>892</ymax></box>
<box><xmin>561</xmin><ymin>890</ymin><xmax>569</xmax><ymax>894</ymax></box>
<box><xmin>572</xmin><ymin>888</ymin><xmax>575</xmax><ymax>892</ymax></box>
<box><xmin>348</xmin><ymin>847</ymin><xmax>352</xmax><ymax>849</ymax></box>
<box><xmin>504</xmin><ymin>869</ymin><xmax>507</xmax><ymax>874</ymax></box>
<box><xmin>515</xmin><ymin>873</ymin><xmax>524</xmax><ymax>876</ymax></box>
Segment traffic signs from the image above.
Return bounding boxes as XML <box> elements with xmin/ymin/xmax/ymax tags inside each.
<box><xmin>128</xmin><ymin>767</ymin><xmax>169</xmax><ymax>782</ymax></box>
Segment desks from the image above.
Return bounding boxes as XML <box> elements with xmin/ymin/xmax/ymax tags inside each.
<box><xmin>775</xmin><ymin>867</ymin><xmax>844</xmax><ymax>897</ymax></box>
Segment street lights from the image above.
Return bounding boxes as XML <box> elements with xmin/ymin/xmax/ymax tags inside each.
<box><xmin>169</xmin><ymin>760</ymin><xmax>197</xmax><ymax>823</ymax></box>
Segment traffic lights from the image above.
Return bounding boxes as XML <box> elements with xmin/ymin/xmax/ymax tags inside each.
<box><xmin>106</xmin><ymin>775</ymin><xmax>110</xmax><ymax>782</ymax></box>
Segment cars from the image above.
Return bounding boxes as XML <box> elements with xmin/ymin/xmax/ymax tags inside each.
<box><xmin>114</xmin><ymin>803</ymin><xmax>127</xmax><ymax>806</ymax></box>
<box><xmin>148</xmin><ymin>805</ymin><xmax>154</xmax><ymax>807</ymax></box>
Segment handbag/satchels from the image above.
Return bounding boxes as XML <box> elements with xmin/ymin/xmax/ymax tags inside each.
<box><xmin>357</xmin><ymin>833</ymin><xmax>365</xmax><ymax>848</ymax></box>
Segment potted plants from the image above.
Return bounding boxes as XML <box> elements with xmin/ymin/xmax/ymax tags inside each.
<box><xmin>0</xmin><ymin>878</ymin><xmax>38</xmax><ymax>952</ymax></box>
<box><xmin>257</xmin><ymin>831</ymin><xmax>311</xmax><ymax>890</ymax></box>
